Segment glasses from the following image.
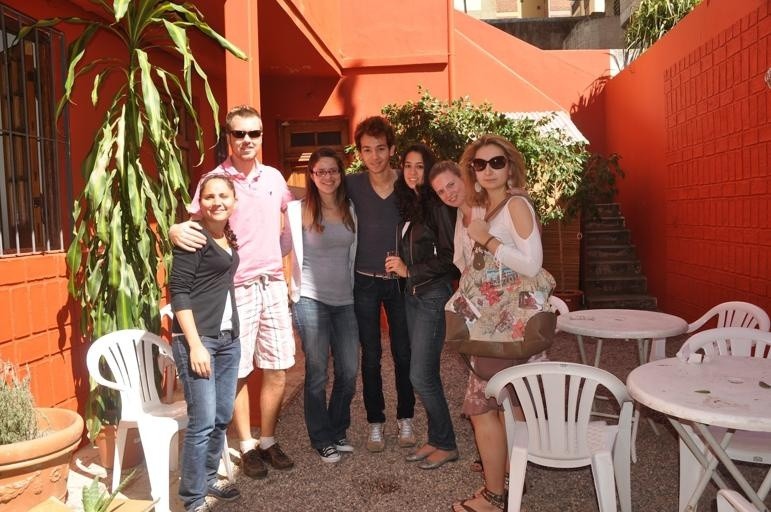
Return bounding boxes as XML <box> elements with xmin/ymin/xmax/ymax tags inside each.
<box><xmin>311</xmin><ymin>168</ymin><xmax>341</xmax><ymax>177</ymax></box>
<box><xmin>472</xmin><ymin>156</ymin><xmax>509</xmax><ymax>172</ymax></box>
<box><xmin>230</xmin><ymin>130</ymin><xmax>261</xmax><ymax>138</ymax></box>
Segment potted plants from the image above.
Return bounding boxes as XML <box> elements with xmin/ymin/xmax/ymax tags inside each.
<box><xmin>536</xmin><ymin>126</ymin><xmax>625</xmax><ymax>312</ymax></box>
<box><xmin>11</xmin><ymin>0</ymin><xmax>249</xmax><ymax>469</ymax></box>
<box><xmin>0</xmin><ymin>360</ymin><xmax>85</xmax><ymax>511</ymax></box>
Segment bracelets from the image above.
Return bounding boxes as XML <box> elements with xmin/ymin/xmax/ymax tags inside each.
<box><xmin>484</xmin><ymin>235</ymin><xmax>496</xmax><ymax>248</ymax></box>
<box><xmin>406</xmin><ymin>265</ymin><xmax>409</xmax><ymax>280</ymax></box>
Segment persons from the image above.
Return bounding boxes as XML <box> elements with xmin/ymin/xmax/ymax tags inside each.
<box><xmin>429</xmin><ymin>160</ymin><xmax>488</xmax><ymax>275</ymax></box>
<box><xmin>346</xmin><ymin>117</ymin><xmax>415</xmax><ymax>452</ymax></box>
<box><xmin>284</xmin><ymin>146</ymin><xmax>357</xmax><ymax>461</ymax></box>
<box><xmin>384</xmin><ymin>145</ymin><xmax>459</xmax><ymax>469</ymax></box>
<box><xmin>445</xmin><ymin>136</ymin><xmax>556</xmax><ymax>512</ymax></box>
<box><xmin>170</xmin><ymin>174</ymin><xmax>241</xmax><ymax>511</ymax></box>
<box><xmin>167</xmin><ymin>104</ymin><xmax>293</xmax><ymax>479</ymax></box>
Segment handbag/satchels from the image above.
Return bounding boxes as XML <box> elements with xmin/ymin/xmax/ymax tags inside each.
<box><xmin>445</xmin><ymin>196</ymin><xmax>557</xmax><ymax>359</ymax></box>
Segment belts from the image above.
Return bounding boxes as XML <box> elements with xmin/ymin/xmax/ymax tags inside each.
<box><xmin>356</xmin><ymin>270</ymin><xmax>397</xmax><ymax>280</ymax></box>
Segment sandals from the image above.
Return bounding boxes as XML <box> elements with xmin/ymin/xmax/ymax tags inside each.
<box><xmin>406</xmin><ymin>444</ymin><xmax>437</xmax><ymax>461</ymax></box>
<box><xmin>452</xmin><ymin>490</ymin><xmax>508</xmax><ymax>512</ymax></box>
<box><xmin>418</xmin><ymin>448</ymin><xmax>458</xmax><ymax>469</ymax></box>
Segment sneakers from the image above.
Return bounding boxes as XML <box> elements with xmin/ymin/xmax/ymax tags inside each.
<box><xmin>396</xmin><ymin>418</ymin><xmax>416</xmax><ymax>447</ymax></box>
<box><xmin>316</xmin><ymin>446</ymin><xmax>341</xmax><ymax>463</ymax></box>
<box><xmin>366</xmin><ymin>422</ymin><xmax>385</xmax><ymax>452</ymax></box>
<box><xmin>332</xmin><ymin>439</ymin><xmax>354</xmax><ymax>452</ymax></box>
<box><xmin>186</xmin><ymin>501</ymin><xmax>211</xmax><ymax>512</ymax></box>
<box><xmin>207</xmin><ymin>481</ymin><xmax>240</xmax><ymax>501</ymax></box>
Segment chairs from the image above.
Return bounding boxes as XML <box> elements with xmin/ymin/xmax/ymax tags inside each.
<box><xmin>484</xmin><ymin>361</ymin><xmax>633</xmax><ymax>512</ymax></box>
<box><xmin>547</xmin><ymin>295</ymin><xmax>569</xmax><ymax>337</ymax></box>
<box><xmin>717</xmin><ymin>488</ymin><xmax>761</xmax><ymax>512</ymax></box>
<box><xmin>676</xmin><ymin>327</ymin><xmax>770</xmax><ymax>510</ymax></box>
<box><xmin>86</xmin><ymin>328</ymin><xmax>236</xmax><ymax>512</ymax></box>
<box><xmin>686</xmin><ymin>301</ymin><xmax>770</xmax><ymax>351</ymax></box>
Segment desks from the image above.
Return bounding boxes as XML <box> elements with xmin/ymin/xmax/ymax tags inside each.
<box><xmin>556</xmin><ymin>308</ymin><xmax>688</xmax><ymax>463</ymax></box>
<box><xmin>625</xmin><ymin>354</ymin><xmax>771</xmax><ymax>512</ymax></box>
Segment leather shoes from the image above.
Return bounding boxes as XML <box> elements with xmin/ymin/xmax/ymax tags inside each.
<box><xmin>240</xmin><ymin>448</ymin><xmax>268</xmax><ymax>479</ymax></box>
<box><xmin>258</xmin><ymin>444</ymin><xmax>294</xmax><ymax>471</ymax></box>
